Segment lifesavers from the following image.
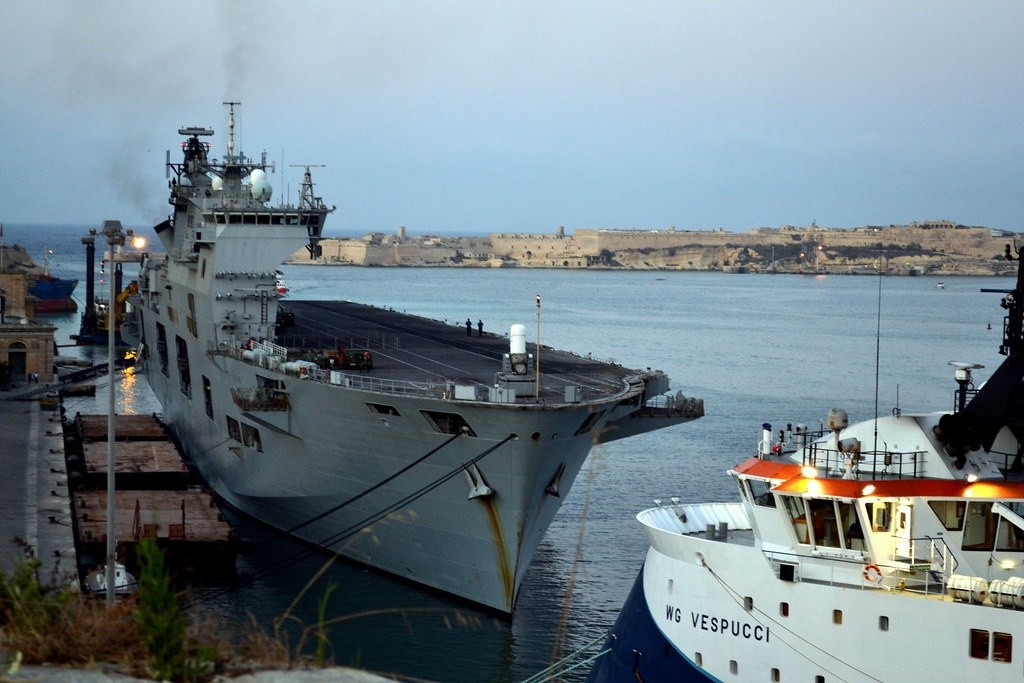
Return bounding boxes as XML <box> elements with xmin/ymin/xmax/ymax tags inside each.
<box><xmin>62</xmin><ymin>415</ymin><xmax>66</xmax><ymax>422</ymax></box>
<box><xmin>864</xmin><ymin>564</ymin><xmax>882</xmax><ymax>582</ymax></box>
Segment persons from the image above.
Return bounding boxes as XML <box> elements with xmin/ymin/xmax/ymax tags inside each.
<box><xmin>466</xmin><ymin>318</ymin><xmax>472</xmax><ymax>335</ymax></box>
<box><xmin>34</xmin><ymin>372</ymin><xmax>39</xmax><ymax>383</ymax></box>
<box><xmin>478</xmin><ymin>320</ymin><xmax>483</xmax><ymax>336</ymax></box>
<box><xmin>28</xmin><ymin>372</ymin><xmax>31</xmax><ymax>384</ymax></box>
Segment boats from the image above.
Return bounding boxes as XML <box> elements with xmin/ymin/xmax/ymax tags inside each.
<box><xmin>274</xmin><ymin>271</ymin><xmax>290</xmax><ymax>297</ymax></box>
<box><xmin>26</xmin><ymin>272</ymin><xmax>80</xmax><ymax>315</ymax></box>
<box><xmin>636</xmin><ymin>232</ymin><xmax>1024</xmax><ymax>683</ymax></box>
<box><xmin>119</xmin><ymin>99</ymin><xmax>705</xmax><ymax>626</ymax></box>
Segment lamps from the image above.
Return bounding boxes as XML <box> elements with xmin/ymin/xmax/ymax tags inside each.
<box><xmin>837</xmin><ymin>437</ymin><xmax>859</xmax><ymax>453</ymax></box>
